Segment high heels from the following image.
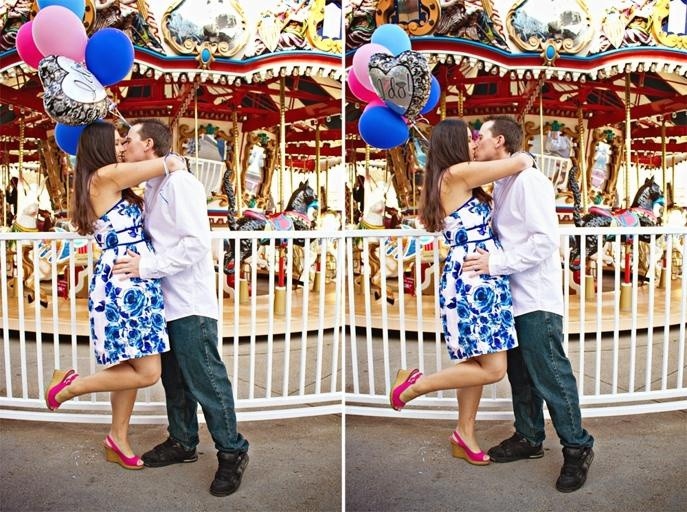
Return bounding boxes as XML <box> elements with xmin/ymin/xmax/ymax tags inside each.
<box><xmin>390</xmin><ymin>368</ymin><xmax>423</xmax><ymax>412</ymax></box>
<box><xmin>101</xmin><ymin>434</ymin><xmax>144</xmax><ymax>470</ymax></box>
<box><xmin>448</xmin><ymin>430</ymin><xmax>490</xmax><ymax>466</ymax></box>
<box><xmin>44</xmin><ymin>369</ymin><xmax>78</xmax><ymax>412</ymax></box>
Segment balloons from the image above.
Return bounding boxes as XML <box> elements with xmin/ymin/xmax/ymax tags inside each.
<box><xmin>11</xmin><ymin>0</ymin><xmax>135</xmax><ymax>158</ymax></box>
<box><xmin>347</xmin><ymin>22</ymin><xmax>442</xmax><ymax>151</ymax></box>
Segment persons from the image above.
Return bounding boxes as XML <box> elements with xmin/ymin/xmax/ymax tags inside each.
<box><xmin>350</xmin><ymin>175</ymin><xmax>364</xmax><ymax>216</ymax></box>
<box><xmin>112</xmin><ymin>119</ymin><xmax>252</xmax><ymax>497</ymax></box>
<box><xmin>458</xmin><ymin>115</ymin><xmax>597</xmax><ymax>494</ymax></box>
<box><xmin>387</xmin><ymin>118</ymin><xmax>537</xmax><ymax>467</ymax></box>
<box><xmin>0</xmin><ymin>165</ymin><xmax>50</xmax><ymax>310</ymax></box>
<box><xmin>41</xmin><ymin>117</ymin><xmax>185</xmax><ymax>474</ymax></box>
<box><xmin>4</xmin><ymin>176</ymin><xmax>18</xmax><ymax>216</ymax></box>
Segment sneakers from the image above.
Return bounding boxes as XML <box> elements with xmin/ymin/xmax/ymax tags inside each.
<box><xmin>210</xmin><ymin>443</ymin><xmax>249</xmax><ymax>497</ymax></box>
<box><xmin>488</xmin><ymin>430</ymin><xmax>544</xmax><ymax>463</ymax></box>
<box><xmin>141</xmin><ymin>437</ymin><xmax>198</xmax><ymax>468</ymax></box>
<box><xmin>556</xmin><ymin>444</ymin><xmax>594</xmax><ymax>493</ymax></box>
